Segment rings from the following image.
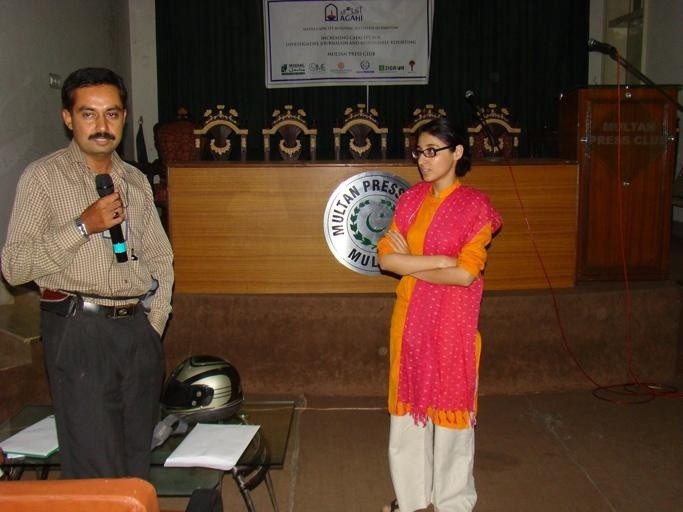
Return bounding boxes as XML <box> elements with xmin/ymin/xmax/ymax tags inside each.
<box><xmin>112</xmin><ymin>209</ymin><xmax>120</xmax><ymax>218</ymax></box>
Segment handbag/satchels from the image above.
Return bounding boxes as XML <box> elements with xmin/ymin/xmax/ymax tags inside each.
<box><xmin>39</xmin><ymin>289</ymin><xmax>78</xmax><ymax>319</ymax></box>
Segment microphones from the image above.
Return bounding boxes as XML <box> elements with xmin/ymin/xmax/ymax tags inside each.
<box><xmin>587</xmin><ymin>38</ymin><xmax>618</xmax><ymax>53</ymax></box>
<box><xmin>465</xmin><ymin>90</ymin><xmax>482</xmax><ymax>116</ymax></box>
<box><xmin>95</xmin><ymin>173</ymin><xmax>130</xmax><ymax>264</ymax></box>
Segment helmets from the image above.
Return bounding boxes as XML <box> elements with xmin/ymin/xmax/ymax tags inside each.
<box><xmin>159</xmin><ymin>355</ymin><xmax>245</xmax><ymax>415</ymax></box>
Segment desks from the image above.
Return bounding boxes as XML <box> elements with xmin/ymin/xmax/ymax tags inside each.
<box><xmin>0</xmin><ymin>403</ymin><xmax>294</xmax><ymax>512</ymax></box>
<box><xmin>164</xmin><ymin>163</ymin><xmax>580</xmax><ymax>293</ymax></box>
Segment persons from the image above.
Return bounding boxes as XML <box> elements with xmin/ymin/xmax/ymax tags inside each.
<box><xmin>376</xmin><ymin>118</ymin><xmax>503</xmax><ymax>512</ymax></box>
<box><xmin>0</xmin><ymin>67</ymin><xmax>175</xmax><ymax>481</ymax></box>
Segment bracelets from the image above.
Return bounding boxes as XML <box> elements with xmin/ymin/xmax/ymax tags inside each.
<box><xmin>74</xmin><ymin>217</ymin><xmax>87</xmax><ymax>237</ymax></box>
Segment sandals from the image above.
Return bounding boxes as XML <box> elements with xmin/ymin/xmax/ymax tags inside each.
<box><xmin>389</xmin><ymin>498</ymin><xmax>400</xmax><ymax>512</ymax></box>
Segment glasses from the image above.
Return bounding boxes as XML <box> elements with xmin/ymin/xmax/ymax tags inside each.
<box><xmin>411</xmin><ymin>145</ymin><xmax>452</xmax><ymax>159</ymax></box>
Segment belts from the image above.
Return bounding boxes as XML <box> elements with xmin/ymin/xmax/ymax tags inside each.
<box><xmin>79</xmin><ymin>301</ymin><xmax>142</xmax><ymax>320</ymax></box>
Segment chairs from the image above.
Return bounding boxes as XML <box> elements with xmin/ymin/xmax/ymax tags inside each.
<box><xmin>136</xmin><ymin>115</ymin><xmax>168</xmax><ymax>209</ymax></box>
<box><xmin>141</xmin><ymin>119</ymin><xmax>201</xmax><ymax>205</ymax></box>
<box><xmin>0</xmin><ymin>476</ymin><xmax>223</xmax><ymax>512</ymax></box>
<box><xmin>403</xmin><ymin>104</ymin><xmax>448</xmax><ymax>159</ymax></box>
<box><xmin>261</xmin><ymin>104</ymin><xmax>319</xmax><ymax>161</ymax></box>
<box><xmin>334</xmin><ymin>103</ymin><xmax>390</xmax><ymax>160</ymax></box>
<box><xmin>468</xmin><ymin>103</ymin><xmax>522</xmax><ymax>160</ymax></box>
<box><xmin>192</xmin><ymin>104</ymin><xmax>249</xmax><ymax>162</ymax></box>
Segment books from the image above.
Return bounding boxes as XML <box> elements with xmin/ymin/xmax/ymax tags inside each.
<box><xmin>163</xmin><ymin>421</ymin><xmax>261</xmax><ymax>471</ymax></box>
<box><xmin>0</xmin><ymin>413</ymin><xmax>59</xmax><ymax>458</ymax></box>
